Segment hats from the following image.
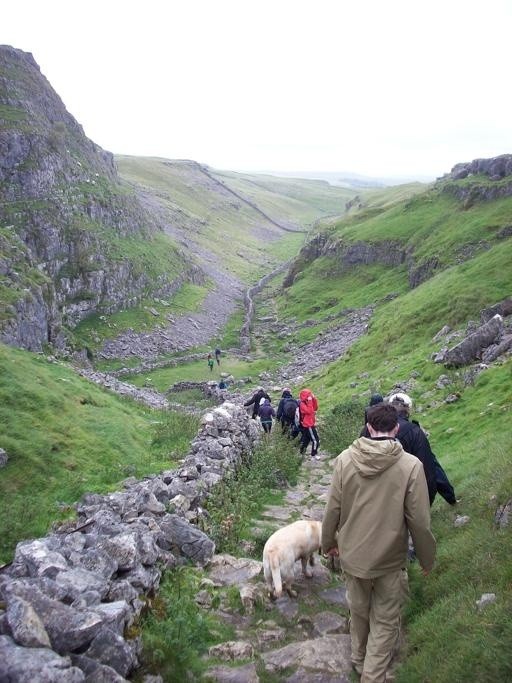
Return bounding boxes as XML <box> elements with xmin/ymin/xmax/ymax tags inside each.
<box><xmin>389</xmin><ymin>393</ymin><xmax>412</xmax><ymax>409</ymax></box>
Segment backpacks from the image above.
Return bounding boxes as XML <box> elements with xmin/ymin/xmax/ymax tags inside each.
<box><xmin>284</xmin><ymin>398</ymin><xmax>297</xmax><ymax>418</ymax></box>
<box><xmin>294</xmin><ymin>400</ymin><xmax>306</xmax><ymax>428</ymax></box>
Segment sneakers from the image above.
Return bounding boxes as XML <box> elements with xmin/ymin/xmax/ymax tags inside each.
<box><xmin>311</xmin><ymin>455</ymin><xmax>321</xmax><ymax>461</ymax></box>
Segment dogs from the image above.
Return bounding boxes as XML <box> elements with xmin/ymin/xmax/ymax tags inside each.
<box><xmin>262</xmin><ymin>519</ymin><xmax>339</xmax><ymax>600</ymax></box>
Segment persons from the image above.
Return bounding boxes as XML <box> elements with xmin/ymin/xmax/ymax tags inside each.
<box><xmin>219</xmin><ymin>379</ymin><xmax>225</xmax><ymax>389</ymax></box>
<box><xmin>359</xmin><ymin>393</ymin><xmax>383</xmax><ymax>438</ymax></box>
<box><xmin>244</xmin><ymin>388</ymin><xmax>319</xmax><ymax>456</ymax></box>
<box><xmin>321</xmin><ymin>402</ymin><xmax>436</xmax><ymax>683</ymax></box>
<box><xmin>359</xmin><ymin>393</ymin><xmax>456</xmax><ymax>509</ymax></box>
<box><xmin>207</xmin><ymin>347</ymin><xmax>220</xmax><ymax>371</ymax></box>
